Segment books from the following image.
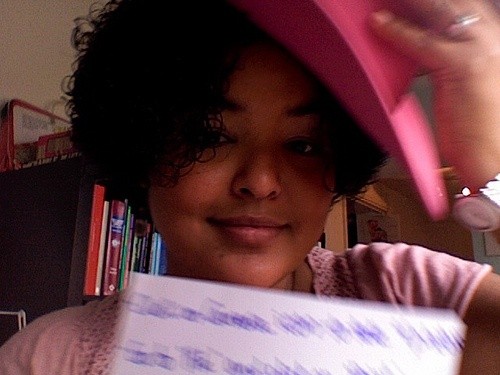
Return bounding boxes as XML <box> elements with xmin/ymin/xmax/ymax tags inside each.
<box><xmin>78</xmin><ymin>175</ymin><xmax>175</xmax><ymax>297</ymax></box>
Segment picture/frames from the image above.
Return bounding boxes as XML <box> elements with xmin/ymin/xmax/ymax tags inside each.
<box><xmin>471</xmin><ymin>230</ymin><xmax>500</xmax><ymax>274</ymax></box>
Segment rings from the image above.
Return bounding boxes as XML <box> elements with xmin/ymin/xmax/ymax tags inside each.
<box><xmin>449</xmin><ymin>12</ymin><xmax>483</xmax><ymax>32</ymax></box>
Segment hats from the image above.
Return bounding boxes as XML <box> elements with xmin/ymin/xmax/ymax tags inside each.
<box><xmin>234</xmin><ymin>0</ymin><xmax>450</xmax><ymax>220</ymax></box>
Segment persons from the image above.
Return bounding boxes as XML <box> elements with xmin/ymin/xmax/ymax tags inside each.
<box><xmin>0</xmin><ymin>0</ymin><xmax>499</xmax><ymax>373</ymax></box>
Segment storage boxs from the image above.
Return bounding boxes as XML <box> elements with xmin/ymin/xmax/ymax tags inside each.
<box><xmin>0</xmin><ymin>97</ymin><xmax>78</xmax><ymax>173</ymax></box>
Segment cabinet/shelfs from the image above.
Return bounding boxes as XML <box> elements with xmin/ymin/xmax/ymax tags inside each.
<box><xmin>0</xmin><ymin>156</ymin><xmax>100</xmax><ymax>347</ymax></box>
